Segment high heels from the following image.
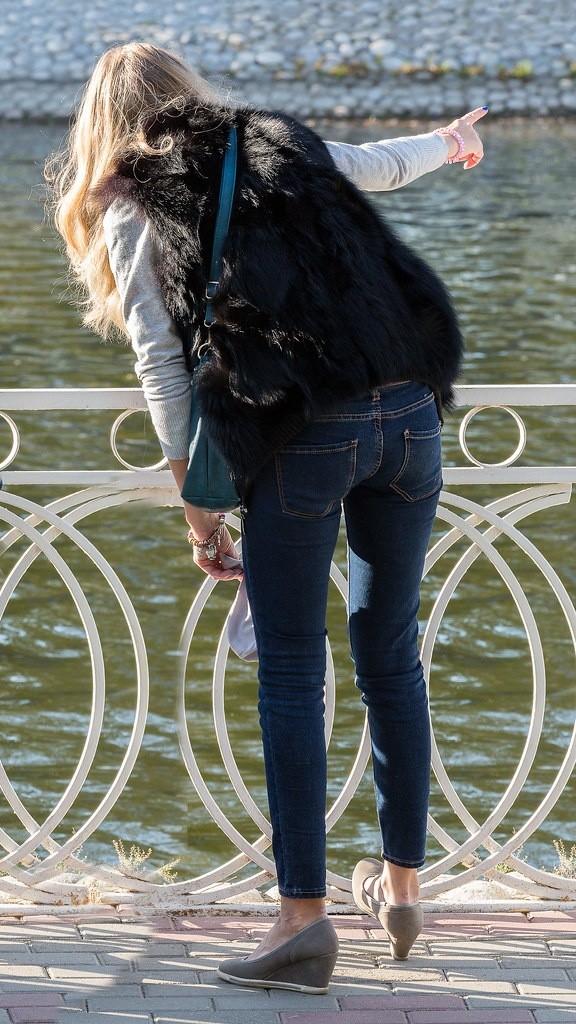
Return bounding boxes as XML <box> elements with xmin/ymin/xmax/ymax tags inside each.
<box><xmin>351</xmin><ymin>857</ymin><xmax>424</xmax><ymax>962</ymax></box>
<box><xmin>217</xmin><ymin>916</ymin><xmax>338</xmax><ymax>994</ymax></box>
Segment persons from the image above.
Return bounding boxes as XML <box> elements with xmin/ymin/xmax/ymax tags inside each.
<box><xmin>49</xmin><ymin>42</ymin><xmax>487</xmax><ymax>995</ymax></box>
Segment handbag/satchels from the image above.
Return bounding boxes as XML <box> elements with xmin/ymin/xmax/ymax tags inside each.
<box><xmin>181</xmin><ymin>357</ymin><xmax>241</xmax><ymax>513</ymax></box>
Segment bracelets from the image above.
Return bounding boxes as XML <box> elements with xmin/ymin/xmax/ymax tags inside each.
<box><xmin>187</xmin><ymin>513</ymin><xmax>224</xmax><ymax>563</ymax></box>
<box><xmin>435</xmin><ymin>128</ymin><xmax>465</xmax><ymax>165</ymax></box>
